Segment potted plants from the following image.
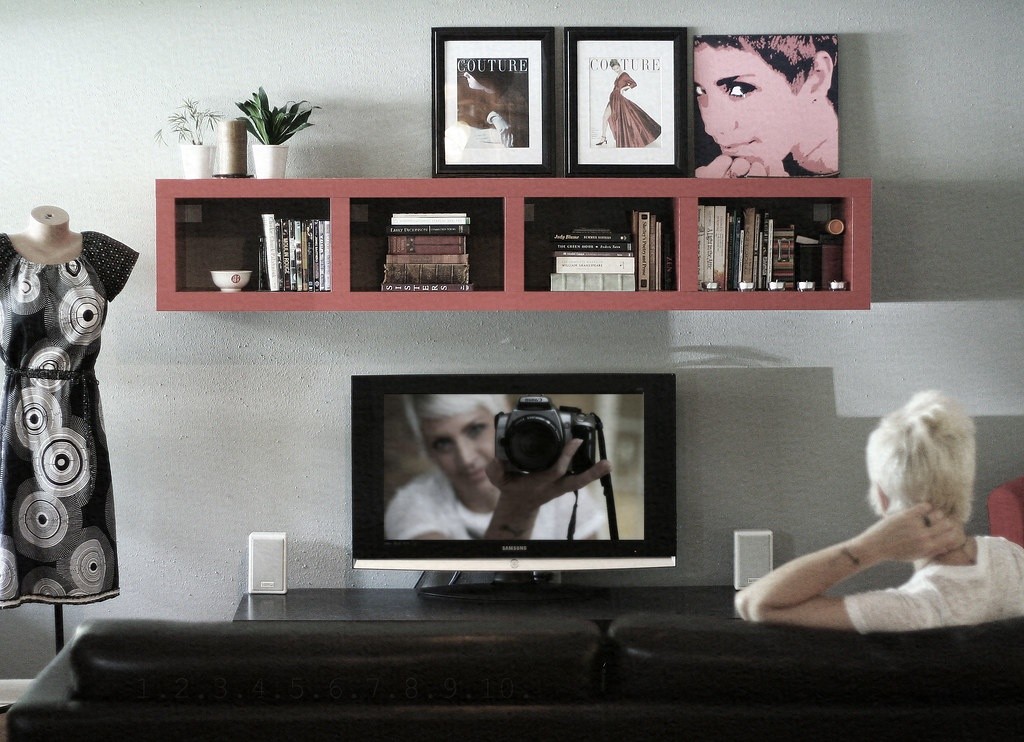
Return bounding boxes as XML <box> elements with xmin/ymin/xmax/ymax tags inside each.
<box><xmin>154</xmin><ymin>97</ymin><xmax>226</xmax><ymax>178</ymax></box>
<box><xmin>236</xmin><ymin>88</ymin><xmax>321</xmax><ymax>178</ymax></box>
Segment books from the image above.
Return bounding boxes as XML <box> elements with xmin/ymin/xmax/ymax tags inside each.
<box><xmin>698</xmin><ymin>204</ymin><xmax>844</xmax><ymax>292</ymax></box>
<box><xmin>247</xmin><ymin>214</ymin><xmax>332</xmax><ymax>292</ymax></box>
<box><xmin>549</xmin><ymin>208</ymin><xmax>676</xmax><ymax>291</ymax></box>
<box><xmin>380</xmin><ymin>213</ymin><xmax>474</xmax><ymax>292</ymax></box>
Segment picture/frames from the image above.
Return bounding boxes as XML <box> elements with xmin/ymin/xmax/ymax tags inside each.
<box><xmin>432</xmin><ymin>26</ymin><xmax>690</xmax><ymax>178</ymax></box>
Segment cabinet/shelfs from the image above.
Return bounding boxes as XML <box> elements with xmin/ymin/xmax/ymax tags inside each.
<box><xmin>154</xmin><ymin>178</ymin><xmax>871</xmax><ymax>310</ymax></box>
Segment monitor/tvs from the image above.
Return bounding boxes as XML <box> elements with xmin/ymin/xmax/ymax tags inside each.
<box><xmin>350</xmin><ymin>374</ymin><xmax>676</xmax><ymax>598</ymax></box>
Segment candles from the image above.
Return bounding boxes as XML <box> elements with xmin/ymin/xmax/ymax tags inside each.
<box><xmin>214</xmin><ymin>119</ymin><xmax>248</xmax><ymax>177</ymax></box>
<box><xmin>702</xmin><ymin>281</ymin><xmax>849</xmax><ymax>291</ymax></box>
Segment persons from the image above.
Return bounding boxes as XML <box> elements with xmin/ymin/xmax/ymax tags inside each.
<box><xmin>0</xmin><ymin>207</ymin><xmax>138</xmax><ymax>610</ymax></box>
<box><xmin>456</xmin><ymin>57</ymin><xmax>530</xmax><ymax>148</ymax></box>
<box><xmin>734</xmin><ymin>391</ymin><xmax>1024</xmax><ymax>635</ymax></box>
<box><xmin>382</xmin><ymin>392</ymin><xmax>614</xmax><ymax>542</ymax></box>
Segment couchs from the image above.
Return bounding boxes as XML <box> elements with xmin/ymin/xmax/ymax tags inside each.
<box><xmin>0</xmin><ymin>618</ymin><xmax>1024</xmax><ymax>742</ymax></box>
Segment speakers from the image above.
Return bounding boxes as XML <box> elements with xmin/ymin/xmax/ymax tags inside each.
<box><xmin>734</xmin><ymin>528</ymin><xmax>774</xmax><ymax>590</ymax></box>
<box><xmin>247</xmin><ymin>532</ymin><xmax>288</xmax><ymax>594</ymax></box>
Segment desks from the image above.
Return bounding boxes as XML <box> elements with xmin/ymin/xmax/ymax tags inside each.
<box><xmin>233</xmin><ymin>583</ymin><xmax>751</xmax><ymax>626</ymax></box>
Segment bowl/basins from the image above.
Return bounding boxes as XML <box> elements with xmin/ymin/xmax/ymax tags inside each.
<box><xmin>210</xmin><ymin>270</ymin><xmax>252</xmax><ymax>292</ymax></box>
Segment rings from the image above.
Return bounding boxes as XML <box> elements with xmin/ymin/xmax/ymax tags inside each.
<box><xmin>923</xmin><ymin>516</ymin><xmax>930</xmax><ymax>527</ymax></box>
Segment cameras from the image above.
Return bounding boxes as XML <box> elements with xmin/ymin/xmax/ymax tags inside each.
<box><xmin>495</xmin><ymin>397</ymin><xmax>596</xmax><ymax>477</ymax></box>
